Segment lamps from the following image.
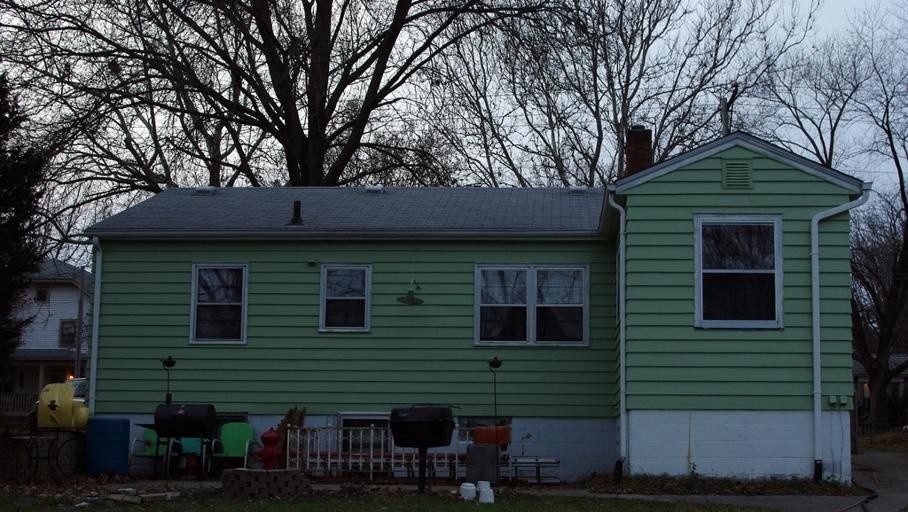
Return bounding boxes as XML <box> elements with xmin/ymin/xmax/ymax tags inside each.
<box><xmin>396</xmin><ymin>278</ymin><xmax>425</xmax><ymax>306</ymax></box>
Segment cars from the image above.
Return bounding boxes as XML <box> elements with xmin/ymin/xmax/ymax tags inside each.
<box><xmin>64</xmin><ymin>377</ymin><xmax>88</xmax><ymax>404</ymax></box>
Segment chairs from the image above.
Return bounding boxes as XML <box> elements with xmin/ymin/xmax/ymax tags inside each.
<box><xmin>126</xmin><ymin>428</ymin><xmax>181</xmax><ymax>477</ymax></box>
<box><xmin>208</xmin><ymin>422</ymin><xmax>255</xmax><ymax>475</ymax></box>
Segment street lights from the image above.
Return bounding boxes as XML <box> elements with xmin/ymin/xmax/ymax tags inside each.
<box><xmin>489</xmin><ymin>355</ymin><xmax>503</xmax><ymax>486</ymax></box>
<box><xmin>160</xmin><ymin>355</ymin><xmax>176</xmax><ymax>403</ymax></box>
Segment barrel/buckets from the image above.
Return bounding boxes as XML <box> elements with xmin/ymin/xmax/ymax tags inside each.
<box><xmin>466</xmin><ymin>438</ymin><xmax>501</xmax><ymax>486</ymax></box>
<box><xmin>84</xmin><ymin>418</ymin><xmax>130</xmax><ymax>476</ymax></box>
